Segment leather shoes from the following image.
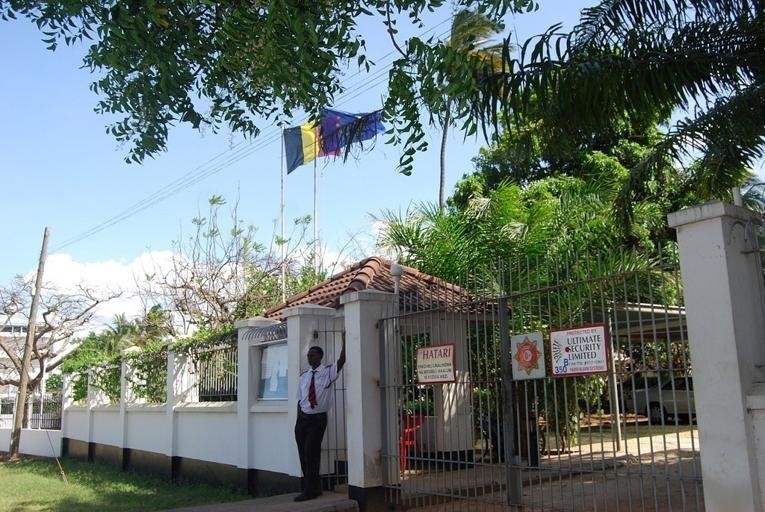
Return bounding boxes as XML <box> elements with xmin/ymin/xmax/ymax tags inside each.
<box><xmin>294</xmin><ymin>492</ymin><xmax>317</xmax><ymax>502</ymax></box>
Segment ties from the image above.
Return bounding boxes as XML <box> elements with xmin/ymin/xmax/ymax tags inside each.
<box><xmin>308</xmin><ymin>371</ymin><xmax>318</xmax><ymax>409</ymax></box>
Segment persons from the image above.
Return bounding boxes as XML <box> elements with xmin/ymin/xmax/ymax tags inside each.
<box><xmin>293</xmin><ymin>328</ymin><xmax>347</xmax><ymax>502</ymax></box>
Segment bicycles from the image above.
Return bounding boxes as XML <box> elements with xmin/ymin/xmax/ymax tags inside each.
<box><xmin>467</xmin><ymin>408</ymin><xmax>548</xmax><ymax>468</ymax></box>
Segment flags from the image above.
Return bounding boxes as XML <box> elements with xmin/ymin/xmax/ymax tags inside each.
<box><xmin>319</xmin><ymin>104</ymin><xmax>384</xmax><ymax>155</ymax></box>
<box><xmin>283</xmin><ymin>116</ymin><xmax>342</xmax><ymax>173</ymax></box>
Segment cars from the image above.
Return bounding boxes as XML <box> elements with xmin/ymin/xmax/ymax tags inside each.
<box><xmin>625</xmin><ymin>374</ymin><xmax>697</xmax><ymax>428</ymax></box>
